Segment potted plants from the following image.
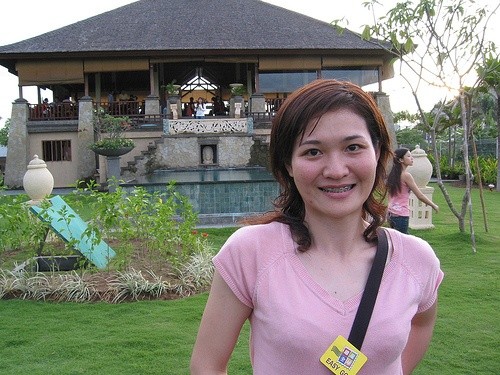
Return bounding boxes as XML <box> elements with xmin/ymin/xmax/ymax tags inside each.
<box><xmin>161</xmin><ymin>79</ymin><xmax>178</xmax><ymax>104</ymax></box>
<box><xmin>231</xmin><ymin>86</ymin><xmax>245</xmax><ymax>103</ymax></box>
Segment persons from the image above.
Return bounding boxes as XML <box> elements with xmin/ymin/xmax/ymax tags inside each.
<box><xmin>184</xmin><ymin>96</ymin><xmax>226</xmax><ymax>119</ymax></box>
<box><xmin>384</xmin><ymin>148</ymin><xmax>439</xmax><ymax>235</ymax></box>
<box><xmin>41</xmin><ymin>90</ymin><xmax>145</xmax><ymax>120</ymax></box>
<box><xmin>272</xmin><ymin>93</ymin><xmax>282</xmax><ymax>111</ymax></box>
<box><xmin>190</xmin><ymin>78</ymin><xmax>444</xmax><ymax>375</ymax></box>
